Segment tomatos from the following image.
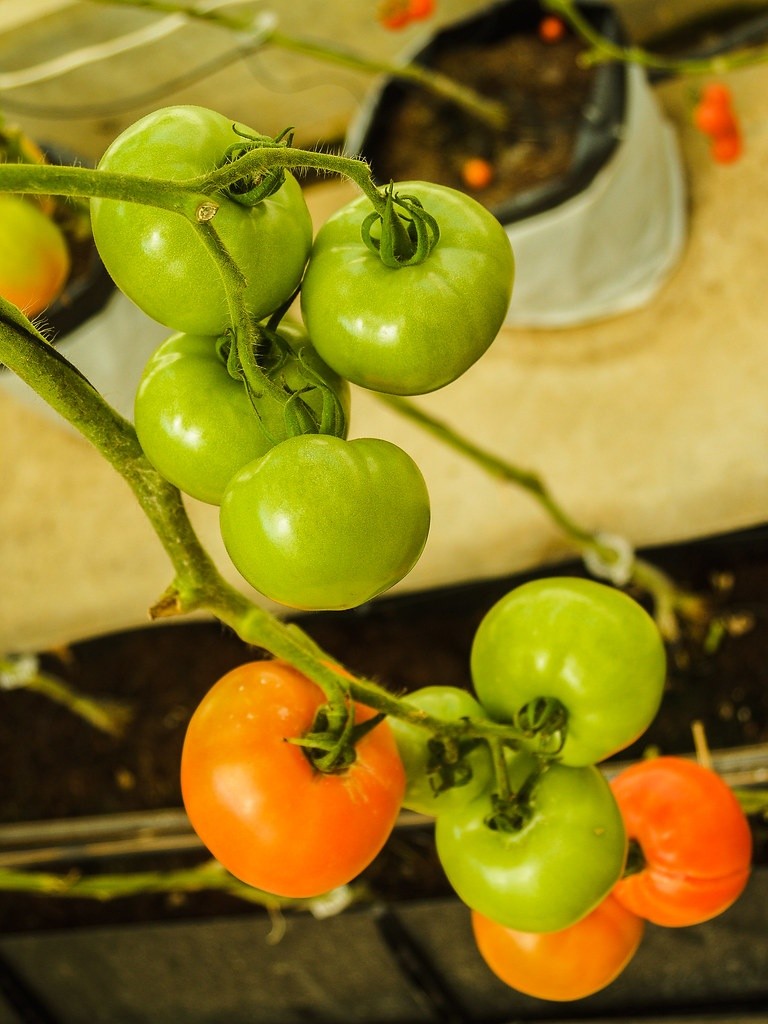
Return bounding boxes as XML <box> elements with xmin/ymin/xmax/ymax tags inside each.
<box><xmin>0</xmin><ymin>105</ymin><xmax>748</xmax><ymax>1002</ymax></box>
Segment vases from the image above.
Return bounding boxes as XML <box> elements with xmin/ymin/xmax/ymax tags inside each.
<box><xmin>341</xmin><ymin>1</ymin><xmax>690</xmax><ymax>331</ymax></box>
